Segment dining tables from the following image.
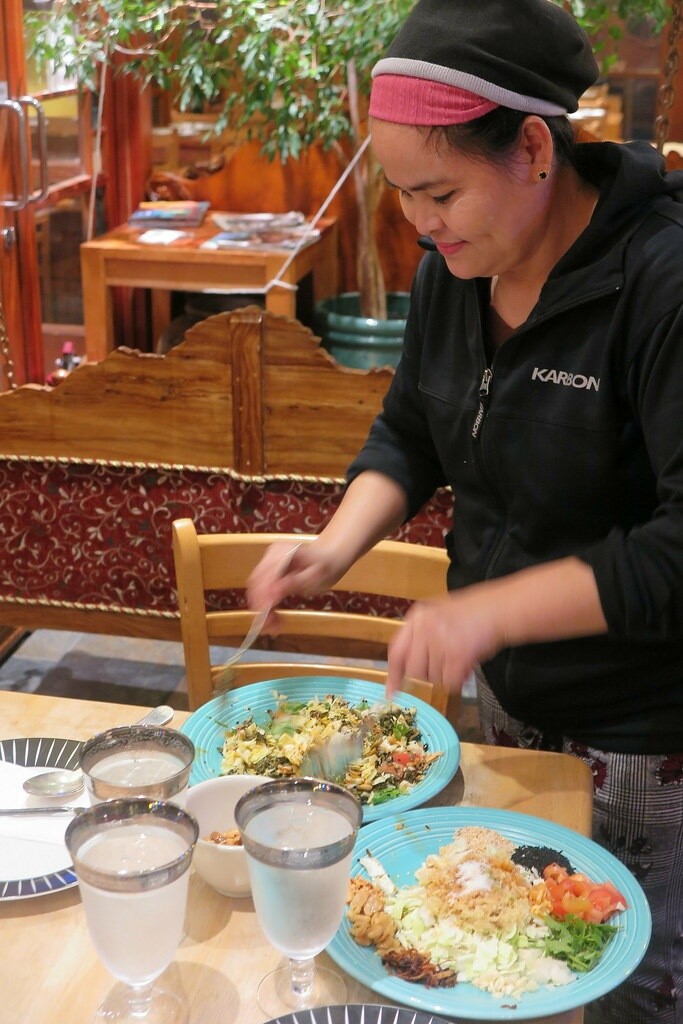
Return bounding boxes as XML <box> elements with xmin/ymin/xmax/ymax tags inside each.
<box><xmin>0</xmin><ymin>687</ymin><xmax>593</xmax><ymax>1024</ymax></box>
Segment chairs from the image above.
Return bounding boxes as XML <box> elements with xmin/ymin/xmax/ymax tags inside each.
<box><xmin>150</xmin><ymin>69</ymin><xmax>322</xmax><ymax>175</ymax></box>
<box><xmin>172</xmin><ymin>516</ymin><xmax>462</xmax><ymax>733</ymax></box>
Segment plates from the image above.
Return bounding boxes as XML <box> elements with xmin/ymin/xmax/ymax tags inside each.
<box><xmin>262</xmin><ymin>1003</ymin><xmax>455</xmax><ymax>1024</ymax></box>
<box><xmin>180</xmin><ymin>675</ymin><xmax>462</xmax><ymax>824</ymax></box>
<box><xmin>323</xmin><ymin>805</ymin><xmax>652</xmax><ymax>1021</ymax></box>
<box><xmin>0</xmin><ymin>737</ymin><xmax>90</xmax><ymax>902</ymax></box>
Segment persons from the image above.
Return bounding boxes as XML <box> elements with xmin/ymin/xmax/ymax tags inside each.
<box><xmin>247</xmin><ymin>0</ymin><xmax>683</xmax><ymax>1024</ymax></box>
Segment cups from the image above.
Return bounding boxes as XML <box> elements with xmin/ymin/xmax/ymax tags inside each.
<box><xmin>80</xmin><ymin>724</ymin><xmax>195</xmax><ymax>814</ymax></box>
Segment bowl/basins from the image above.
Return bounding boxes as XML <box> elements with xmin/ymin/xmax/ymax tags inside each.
<box><xmin>186</xmin><ymin>773</ymin><xmax>283</xmax><ymax>898</ymax></box>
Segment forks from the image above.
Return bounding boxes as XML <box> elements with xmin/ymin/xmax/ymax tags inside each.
<box><xmin>213</xmin><ymin>541</ymin><xmax>303</xmax><ymax>714</ymax></box>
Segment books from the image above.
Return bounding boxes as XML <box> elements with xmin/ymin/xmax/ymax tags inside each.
<box><xmin>131</xmin><ymin>197</ymin><xmax>209</xmax><ymax>229</ymax></box>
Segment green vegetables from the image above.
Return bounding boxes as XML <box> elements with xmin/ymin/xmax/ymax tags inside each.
<box><xmin>529</xmin><ymin>915</ymin><xmax>624</xmax><ymax>970</ymax></box>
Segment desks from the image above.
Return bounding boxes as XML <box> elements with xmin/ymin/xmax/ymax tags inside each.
<box><xmin>78</xmin><ymin>208</ymin><xmax>340</xmax><ymax>364</ymax></box>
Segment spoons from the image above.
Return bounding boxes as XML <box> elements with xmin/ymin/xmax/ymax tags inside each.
<box><xmin>23</xmin><ymin>704</ymin><xmax>176</xmax><ymax>800</ymax></box>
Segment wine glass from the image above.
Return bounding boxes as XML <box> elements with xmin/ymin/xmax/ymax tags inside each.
<box><xmin>235</xmin><ymin>776</ymin><xmax>363</xmax><ymax>1020</ymax></box>
<box><xmin>64</xmin><ymin>797</ymin><xmax>199</xmax><ymax>1024</ymax></box>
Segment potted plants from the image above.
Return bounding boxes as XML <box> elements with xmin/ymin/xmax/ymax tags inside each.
<box><xmin>23</xmin><ymin>0</ymin><xmax>676</xmax><ymax>371</ymax></box>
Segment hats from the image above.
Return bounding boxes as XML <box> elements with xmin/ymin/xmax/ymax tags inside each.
<box><xmin>365</xmin><ymin>0</ymin><xmax>602</xmax><ymax>126</ymax></box>
